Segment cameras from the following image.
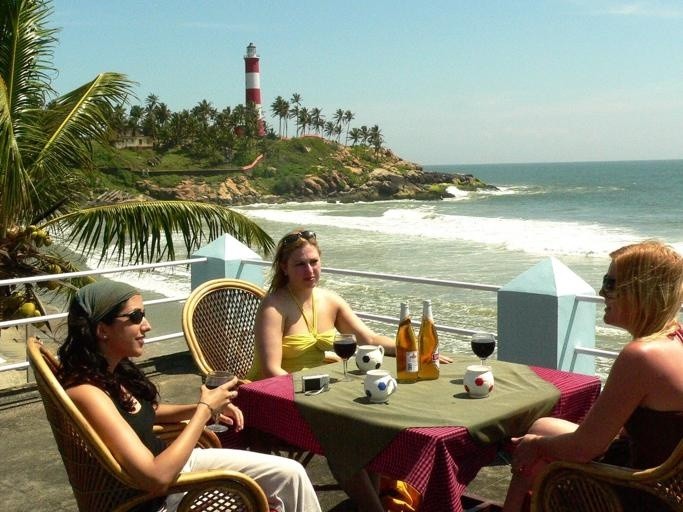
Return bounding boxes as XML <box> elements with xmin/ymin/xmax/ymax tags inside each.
<box><xmin>302</xmin><ymin>375</ymin><xmax>329</xmax><ymax>392</ymax></box>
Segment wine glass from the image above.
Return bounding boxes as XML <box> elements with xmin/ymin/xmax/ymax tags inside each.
<box><xmin>205</xmin><ymin>371</ymin><xmax>237</xmax><ymax>432</ymax></box>
<box><xmin>333</xmin><ymin>334</ymin><xmax>359</xmax><ymax>383</ymax></box>
<box><xmin>470</xmin><ymin>333</ymin><xmax>495</xmax><ymax>365</ymax></box>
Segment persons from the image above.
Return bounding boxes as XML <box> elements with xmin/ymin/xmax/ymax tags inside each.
<box><xmin>501</xmin><ymin>239</ymin><xmax>683</xmax><ymax>511</ymax></box>
<box><xmin>242</xmin><ymin>229</ymin><xmax>454</xmax><ymax>512</ymax></box>
<box><xmin>55</xmin><ymin>279</ymin><xmax>322</xmax><ymax>512</ymax></box>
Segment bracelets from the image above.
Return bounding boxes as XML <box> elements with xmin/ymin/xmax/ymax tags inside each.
<box><xmin>196</xmin><ymin>400</ymin><xmax>218</xmax><ymax>421</ymax></box>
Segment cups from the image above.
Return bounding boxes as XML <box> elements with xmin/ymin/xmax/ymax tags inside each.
<box><xmin>362</xmin><ymin>369</ymin><xmax>397</xmax><ymax>404</ymax></box>
<box><xmin>463</xmin><ymin>365</ymin><xmax>494</xmax><ymax>399</ymax></box>
<box><xmin>357</xmin><ymin>345</ymin><xmax>384</xmax><ymax>373</ymax></box>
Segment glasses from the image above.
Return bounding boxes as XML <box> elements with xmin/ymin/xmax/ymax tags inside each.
<box><xmin>111</xmin><ymin>308</ymin><xmax>147</xmax><ymax>324</ymax></box>
<box><xmin>282</xmin><ymin>231</ymin><xmax>317</xmax><ymax>245</ymax></box>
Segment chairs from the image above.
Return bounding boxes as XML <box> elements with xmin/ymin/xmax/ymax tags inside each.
<box><xmin>182</xmin><ymin>278</ymin><xmax>269</xmax><ymax>384</ymax></box>
<box><xmin>529</xmin><ymin>434</ymin><xmax>683</xmax><ymax>511</ymax></box>
<box><xmin>26</xmin><ymin>337</ymin><xmax>271</xmax><ymax>512</ymax></box>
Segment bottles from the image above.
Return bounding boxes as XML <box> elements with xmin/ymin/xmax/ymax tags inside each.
<box><xmin>418</xmin><ymin>299</ymin><xmax>440</xmax><ymax>380</ymax></box>
<box><xmin>396</xmin><ymin>302</ymin><xmax>420</xmax><ymax>383</ymax></box>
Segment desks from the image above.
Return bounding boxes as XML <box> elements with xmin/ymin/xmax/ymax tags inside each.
<box><xmin>236</xmin><ymin>351</ymin><xmax>601</xmax><ymax>512</ymax></box>
